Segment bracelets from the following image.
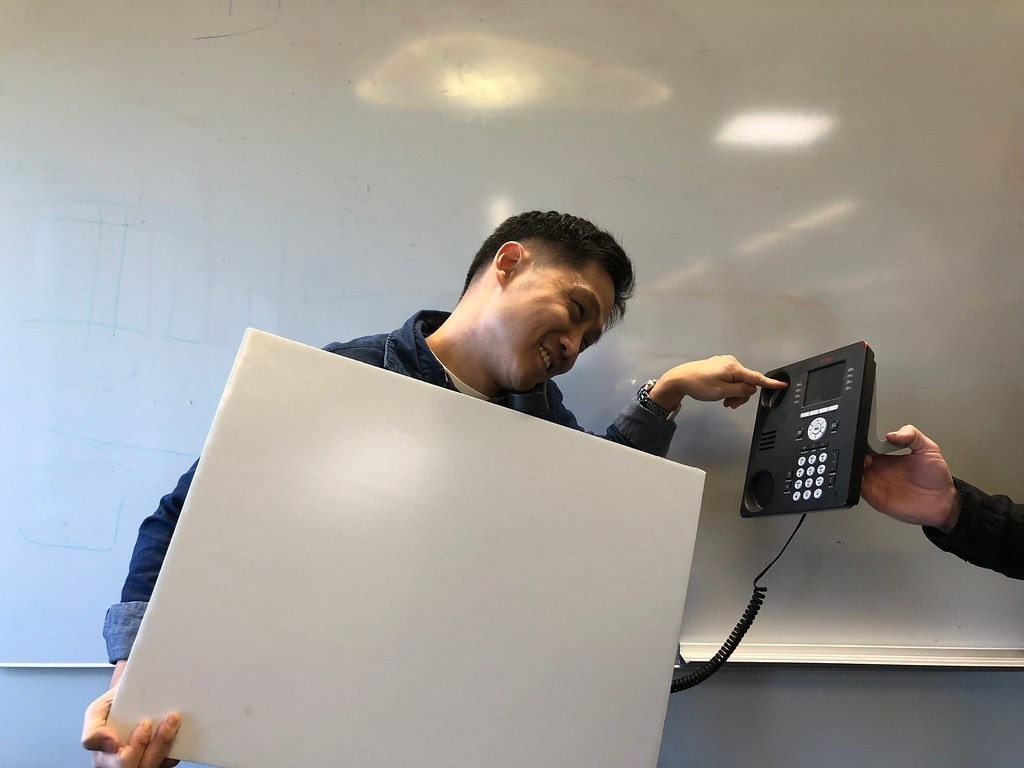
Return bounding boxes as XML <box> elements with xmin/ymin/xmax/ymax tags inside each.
<box><xmin>638</xmin><ymin>380</ymin><xmax>681</xmax><ymax>419</ymax></box>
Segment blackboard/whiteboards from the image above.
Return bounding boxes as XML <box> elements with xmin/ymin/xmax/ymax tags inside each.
<box><xmin>0</xmin><ymin>2</ymin><xmax>1024</xmax><ymax>668</ymax></box>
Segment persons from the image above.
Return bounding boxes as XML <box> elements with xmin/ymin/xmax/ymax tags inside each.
<box><xmin>81</xmin><ymin>211</ymin><xmax>789</xmax><ymax>768</ymax></box>
<box><xmin>860</xmin><ymin>425</ymin><xmax>1024</xmax><ymax>581</ymax></box>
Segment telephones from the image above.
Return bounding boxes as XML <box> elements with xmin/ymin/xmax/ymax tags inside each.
<box><xmin>509</xmin><ymin>379</ymin><xmax>549</xmax><ymax>414</ymax></box>
